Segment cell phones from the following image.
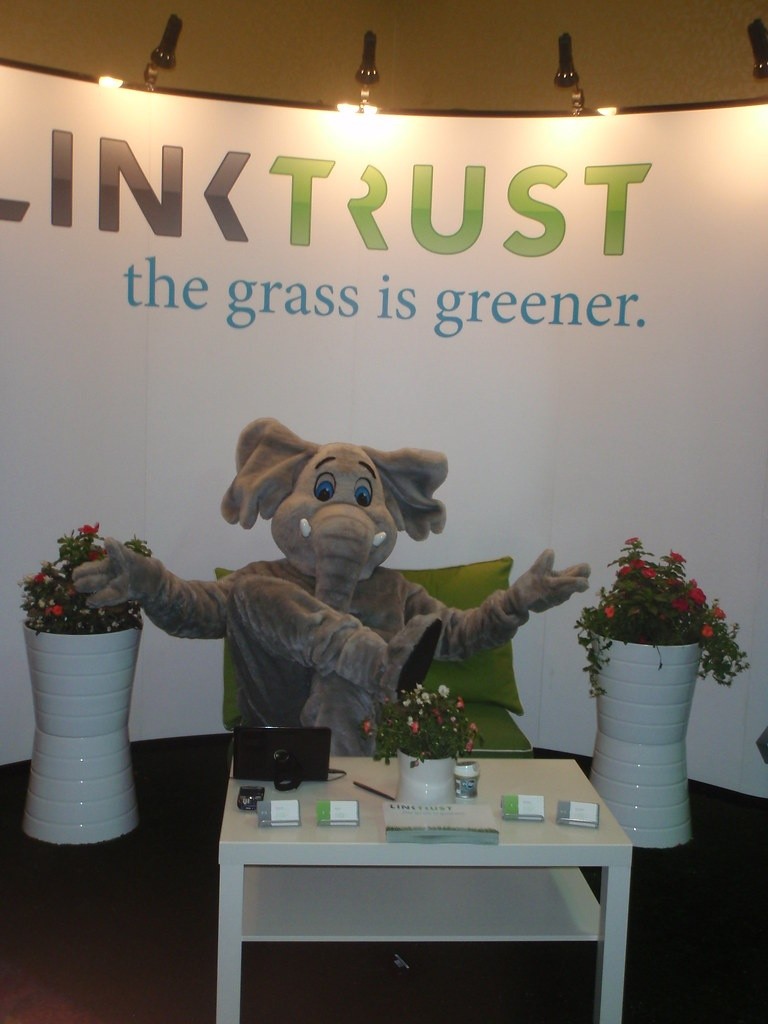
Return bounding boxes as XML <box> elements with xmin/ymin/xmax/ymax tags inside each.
<box><xmin>237</xmin><ymin>786</ymin><xmax>264</xmax><ymax>810</ymax></box>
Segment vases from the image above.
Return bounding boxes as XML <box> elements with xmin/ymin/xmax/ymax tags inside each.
<box><xmin>21</xmin><ymin>621</ymin><xmax>143</xmax><ymax>844</ymax></box>
<box><xmin>399</xmin><ymin>749</ymin><xmax>455</xmax><ymax>790</ymax></box>
<box><xmin>586</xmin><ymin>628</ymin><xmax>702</xmax><ymax>852</ymax></box>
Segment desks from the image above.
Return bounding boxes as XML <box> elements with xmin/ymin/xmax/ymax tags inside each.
<box><xmin>218</xmin><ymin>755</ymin><xmax>633</xmax><ymax>1024</ymax></box>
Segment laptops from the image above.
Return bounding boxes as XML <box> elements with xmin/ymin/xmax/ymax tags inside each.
<box><xmin>234</xmin><ymin>724</ymin><xmax>332</xmax><ymax>783</ymax></box>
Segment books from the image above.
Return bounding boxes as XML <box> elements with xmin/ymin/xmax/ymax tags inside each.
<box><xmin>382</xmin><ymin>800</ymin><xmax>500</xmax><ymax>846</ymax></box>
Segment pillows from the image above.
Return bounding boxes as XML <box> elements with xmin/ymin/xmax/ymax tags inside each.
<box><xmin>216</xmin><ymin>562</ymin><xmax>524</xmax><ymax>730</ymax></box>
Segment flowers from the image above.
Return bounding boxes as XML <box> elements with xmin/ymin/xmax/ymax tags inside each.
<box><xmin>17</xmin><ymin>522</ymin><xmax>154</xmax><ymax>636</ymax></box>
<box><xmin>363</xmin><ymin>680</ymin><xmax>480</xmax><ymax>767</ymax></box>
<box><xmin>569</xmin><ymin>536</ymin><xmax>752</xmax><ymax>697</ymax></box>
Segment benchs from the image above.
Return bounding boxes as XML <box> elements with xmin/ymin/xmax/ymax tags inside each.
<box><xmin>227</xmin><ymin>701</ymin><xmax>532</xmax><ymax>778</ymax></box>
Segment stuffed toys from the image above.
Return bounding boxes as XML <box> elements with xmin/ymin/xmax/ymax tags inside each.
<box><xmin>73</xmin><ymin>421</ymin><xmax>591</xmax><ymax>757</ymax></box>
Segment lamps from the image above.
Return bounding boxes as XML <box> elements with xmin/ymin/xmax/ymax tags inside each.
<box><xmin>143</xmin><ymin>14</ymin><xmax>183</xmax><ymax>92</ymax></box>
<box><xmin>551</xmin><ymin>32</ymin><xmax>583</xmax><ymax>117</ymax></box>
<box><xmin>353</xmin><ymin>30</ymin><xmax>379</xmax><ymax>111</ymax></box>
<box><xmin>746</xmin><ymin>18</ymin><xmax>768</xmax><ymax>79</ymax></box>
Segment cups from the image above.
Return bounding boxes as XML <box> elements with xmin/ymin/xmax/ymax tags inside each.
<box><xmin>454</xmin><ymin>761</ymin><xmax>480</xmax><ymax>799</ymax></box>
